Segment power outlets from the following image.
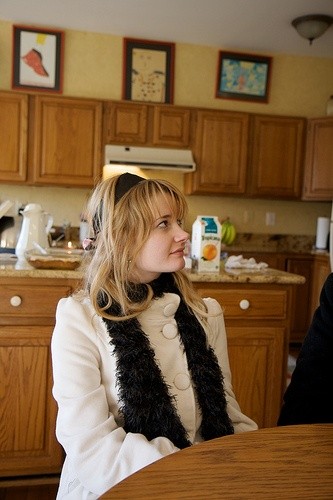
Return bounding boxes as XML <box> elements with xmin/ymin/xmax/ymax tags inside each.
<box><xmin>266</xmin><ymin>210</ymin><xmax>275</xmax><ymax>225</ymax></box>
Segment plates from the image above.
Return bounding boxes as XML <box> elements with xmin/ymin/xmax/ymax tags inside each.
<box><xmin>27</xmin><ymin>255</ymin><xmax>82</xmax><ymax>271</ymax></box>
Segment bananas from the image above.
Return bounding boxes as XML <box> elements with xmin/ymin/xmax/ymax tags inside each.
<box><xmin>220</xmin><ymin>218</ymin><xmax>235</xmax><ymax>245</ymax></box>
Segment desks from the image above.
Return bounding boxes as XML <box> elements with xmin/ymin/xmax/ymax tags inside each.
<box><xmin>98</xmin><ymin>424</ymin><xmax>333</xmax><ymax>500</ymax></box>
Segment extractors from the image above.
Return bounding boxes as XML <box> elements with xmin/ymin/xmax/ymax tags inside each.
<box><xmin>104</xmin><ymin>144</ymin><xmax>196</xmax><ymax>174</ymax></box>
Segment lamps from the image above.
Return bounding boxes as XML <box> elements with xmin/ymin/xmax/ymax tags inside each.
<box><xmin>292</xmin><ymin>14</ymin><xmax>333</xmax><ymax>46</ymax></box>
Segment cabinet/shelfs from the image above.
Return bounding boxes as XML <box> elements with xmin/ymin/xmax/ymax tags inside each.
<box><xmin>0</xmin><ymin>276</ymin><xmax>82</xmax><ymax>486</ymax></box>
<box><xmin>303</xmin><ymin>117</ymin><xmax>333</xmax><ymax>202</ymax></box>
<box><xmin>194</xmin><ymin>281</ymin><xmax>297</xmax><ymax>427</ymax></box>
<box><xmin>283</xmin><ymin>255</ymin><xmax>312</xmax><ymax>344</ymax></box>
<box><xmin>104</xmin><ymin>99</ymin><xmax>192</xmax><ymax>146</ymax></box>
<box><xmin>190</xmin><ymin>108</ymin><xmax>250</xmax><ymax>196</ymax></box>
<box><xmin>309</xmin><ymin>255</ymin><xmax>331</xmax><ymax>325</ymax></box>
<box><xmin>0</xmin><ymin>90</ymin><xmax>28</xmax><ymax>182</ymax></box>
<box><xmin>31</xmin><ymin>93</ymin><xmax>101</xmax><ymax>185</ymax></box>
<box><xmin>248</xmin><ymin>114</ymin><xmax>305</xmax><ymax>199</ymax></box>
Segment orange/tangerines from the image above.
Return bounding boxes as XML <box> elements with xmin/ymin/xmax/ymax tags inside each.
<box><xmin>203</xmin><ymin>244</ymin><xmax>217</xmax><ymax>260</ymax></box>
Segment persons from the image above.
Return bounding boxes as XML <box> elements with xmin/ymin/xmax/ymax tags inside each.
<box><xmin>277</xmin><ymin>273</ymin><xmax>333</xmax><ymax>426</ymax></box>
<box><xmin>51</xmin><ymin>173</ymin><xmax>258</xmax><ymax>500</ymax></box>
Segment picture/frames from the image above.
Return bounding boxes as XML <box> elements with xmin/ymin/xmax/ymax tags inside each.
<box><xmin>11</xmin><ymin>24</ymin><xmax>65</xmax><ymax>95</ymax></box>
<box><xmin>214</xmin><ymin>50</ymin><xmax>272</xmax><ymax>104</ymax></box>
<box><xmin>122</xmin><ymin>35</ymin><xmax>175</xmax><ymax>103</ymax></box>
